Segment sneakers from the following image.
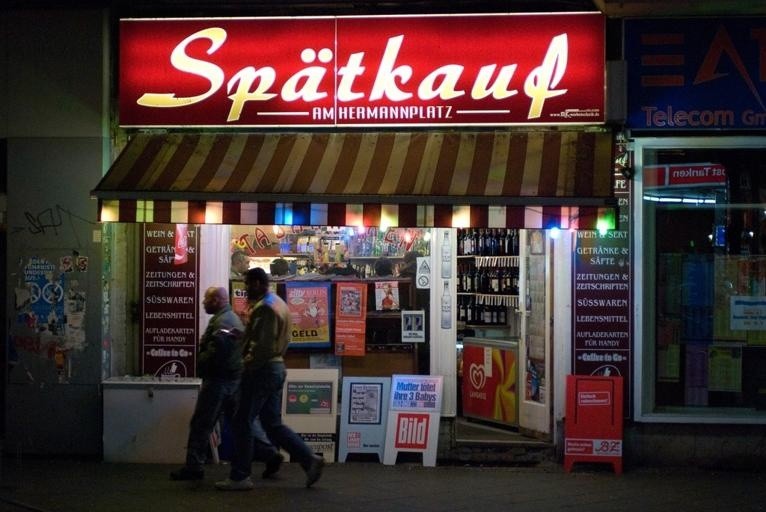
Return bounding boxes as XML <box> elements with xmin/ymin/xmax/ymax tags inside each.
<box><xmin>262</xmin><ymin>453</ymin><xmax>284</xmax><ymax>479</ymax></box>
<box><xmin>169</xmin><ymin>467</ymin><xmax>206</xmax><ymax>479</ymax></box>
<box><xmin>304</xmin><ymin>454</ymin><xmax>325</xmax><ymax>488</ymax></box>
<box><xmin>215</xmin><ymin>475</ymin><xmax>254</xmax><ymax>490</ymax></box>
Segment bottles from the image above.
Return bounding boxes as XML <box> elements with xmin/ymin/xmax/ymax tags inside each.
<box><xmin>323</xmin><ymin>241</ymin><xmax>340</xmax><ymax>265</ymax></box>
<box><xmin>458</xmin><ymin>228</ymin><xmax>519</xmax><ymax>325</ymax></box>
<box><xmin>441</xmin><ymin>232</ymin><xmax>452</xmax><ymax>329</ymax></box>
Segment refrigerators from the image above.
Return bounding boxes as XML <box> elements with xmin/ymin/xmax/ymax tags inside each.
<box><xmin>518</xmin><ymin>229</ymin><xmax>571</xmax><ymax>447</ymax></box>
<box><xmin>461</xmin><ymin>337</ymin><xmax>519</xmax><ymax>430</ymax></box>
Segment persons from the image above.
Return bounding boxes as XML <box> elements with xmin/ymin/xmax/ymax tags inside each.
<box><xmin>169</xmin><ymin>286</ymin><xmax>284</xmax><ymax>480</ymax></box>
<box><xmin>231</xmin><ymin>251</ymin><xmax>250</xmax><ymax>279</ymax></box>
<box><xmin>379</xmin><ymin>282</ymin><xmax>399</xmax><ymax>310</ymax></box>
<box><xmin>213</xmin><ymin>267</ymin><xmax>325</xmax><ymax>491</ymax></box>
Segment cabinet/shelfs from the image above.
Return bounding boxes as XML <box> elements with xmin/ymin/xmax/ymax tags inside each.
<box><xmin>228</xmin><ymin>273</ymin><xmax>415</xmax><ymax>377</ymax></box>
<box><xmin>457</xmin><ymin>255</ymin><xmax>514</xmax><ymax>329</ymax></box>
